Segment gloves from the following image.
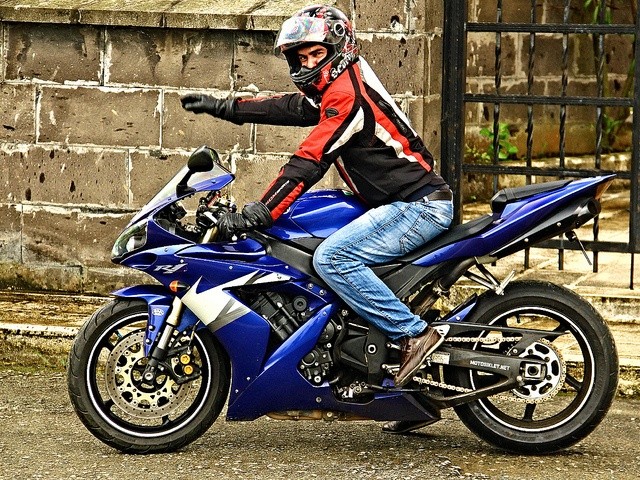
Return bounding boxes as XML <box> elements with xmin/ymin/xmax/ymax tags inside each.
<box><xmin>180</xmin><ymin>94</ymin><xmax>243</xmax><ymax>125</ymax></box>
<box><xmin>216</xmin><ymin>200</ymin><xmax>273</xmax><ymax>241</ymax></box>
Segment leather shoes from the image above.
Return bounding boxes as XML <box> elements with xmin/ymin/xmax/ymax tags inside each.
<box><xmin>394</xmin><ymin>325</ymin><xmax>444</xmax><ymax>387</ymax></box>
<box><xmin>382</xmin><ymin>409</ymin><xmax>440</xmax><ymax>434</ymax></box>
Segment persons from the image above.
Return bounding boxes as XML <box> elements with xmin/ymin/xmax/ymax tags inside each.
<box><xmin>180</xmin><ymin>4</ymin><xmax>455</xmax><ymax>433</ymax></box>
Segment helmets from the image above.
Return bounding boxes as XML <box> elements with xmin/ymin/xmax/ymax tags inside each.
<box><xmin>273</xmin><ymin>3</ymin><xmax>359</xmax><ymax>99</ymax></box>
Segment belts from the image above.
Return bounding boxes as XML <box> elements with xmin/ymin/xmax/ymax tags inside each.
<box><xmin>417</xmin><ymin>192</ymin><xmax>451</xmax><ymax>202</ymax></box>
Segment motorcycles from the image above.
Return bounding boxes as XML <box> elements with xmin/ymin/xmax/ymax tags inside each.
<box><xmin>68</xmin><ymin>145</ymin><xmax>619</xmax><ymax>454</ymax></box>
